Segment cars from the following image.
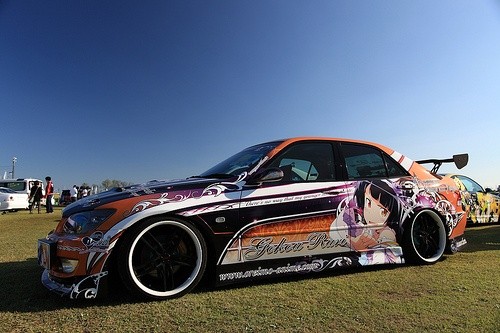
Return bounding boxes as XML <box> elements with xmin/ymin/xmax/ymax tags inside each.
<box><xmin>0</xmin><ymin>187</ymin><xmax>29</xmax><ymax>210</ymax></box>
<box><xmin>59</xmin><ymin>190</ymin><xmax>82</xmax><ymax>207</ymax></box>
<box><xmin>437</xmin><ymin>173</ymin><xmax>500</xmax><ymax>223</ymax></box>
<box><xmin>37</xmin><ymin>138</ymin><xmax>472</xmax><ymax>302</ymax></box>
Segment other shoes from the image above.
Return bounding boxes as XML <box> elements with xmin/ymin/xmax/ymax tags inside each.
<box><xmin>38</xmin><ymin>212</ymin><xmax>42</xmax><ymax>214</ymax></box>
<box><xmin>29</xmin><ymin>212</ymin><xmax>33</xmax><ymax>214</ymax></box>
<box><xmin>46</xmin><ymin>212</ymin><xmax>51</xmax><ymax>214</ymax></box>
<box><xmin>51</xmin><ymin>212</ymin><xmax>54</xmax><ymax>213</ymax></box>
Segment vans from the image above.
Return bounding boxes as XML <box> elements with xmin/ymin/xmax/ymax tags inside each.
<box><xmin>0</xmin><ymin>179</ymin><xmax>46</xmax><ymax>206</ymax></box>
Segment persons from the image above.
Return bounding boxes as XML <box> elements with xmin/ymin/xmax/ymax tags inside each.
<box><xmin>29</xmin><ymin>180</ymin><xmax>43</xmax><ymax>214</ymax></box>
<box><xmin>70</xmin><ymin>185</ymin><xmax>89</xmax><ymax>203</ymax></box>
<box><xmin>43</xmin><ymin>176</ymin><xmax>55</xmax><ymax>213</ymax></box>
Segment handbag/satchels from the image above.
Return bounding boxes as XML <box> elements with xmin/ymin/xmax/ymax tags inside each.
<box><xmin>28</xmin><ymin>196</ymin><xmax>34</xmax><ymax>203</ymax></box>
